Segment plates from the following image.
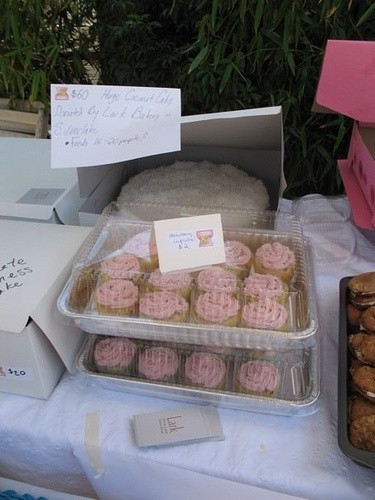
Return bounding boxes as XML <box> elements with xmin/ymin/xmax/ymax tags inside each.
<box><xmin>338</xmin><ymin>276</ymin><xmax>375</xmax><ymax>470</ymax></box>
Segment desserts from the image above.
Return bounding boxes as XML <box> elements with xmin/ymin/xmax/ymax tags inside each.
<box><xmin>92</xmin><ymin>242</ymin><xmax>297</xmax><ymax>395</ymax></box>
<box><xmin>345</xmin><ymin>271</ymin><xmax>375</xmax><ymax>453</ymax></box>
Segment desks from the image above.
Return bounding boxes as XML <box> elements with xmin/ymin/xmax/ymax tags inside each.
<box><xmin>0</xmin><ymin>198</ymin><xmax>374</xmax><ymax>500</ymax></box>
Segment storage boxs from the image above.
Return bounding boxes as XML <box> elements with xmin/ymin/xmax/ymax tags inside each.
<box><xmin>0</xmin><ymin>220</ymin><xmax>94</xmax><ymax>400</ymax></box>
<box><xmin>57</xmin><ymin>199</ymin><xmax>322</xmax><ymax>349</ymax></box>
<box><xmin>77</xmin><ymin>106</ymin><xmax>288</xmax><ymax>229</ymax></box>
<box><xmin>310</xmin><ymin>39</ymin><xmax>374</xmax><ymax>232</ymax></box>
<box><xmin>1</xmin><ymin>136</ymin><xmax>81</xmax><ymax>227</ymax></box>
<box><xmin>75</xmin><ymin>334</ymin><xmax>324</xmax><ymax>415</ymax></box>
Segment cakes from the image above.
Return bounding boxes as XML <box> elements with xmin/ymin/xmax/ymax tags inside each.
<box><xmin>116</xmin><ymin>159</ymin><xmax>269</xmax><ymax>228</ymax></box>
